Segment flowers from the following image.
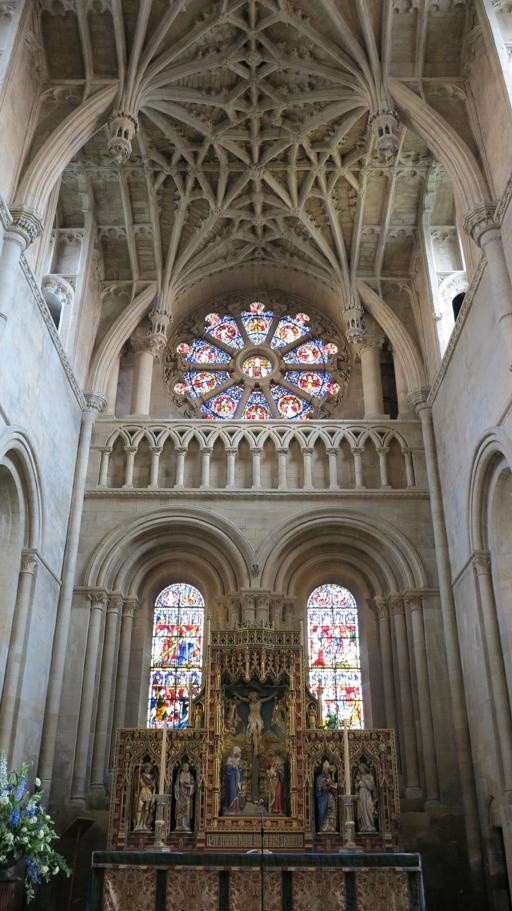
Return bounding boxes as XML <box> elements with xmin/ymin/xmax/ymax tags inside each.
<box><xmin>0</xmin><ymin>751</ymin><xmax>71</xmax><ymax>904</ymax></box>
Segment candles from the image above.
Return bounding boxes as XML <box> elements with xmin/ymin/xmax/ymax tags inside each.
<box><xmin>158</xmin><ymin>718</ymin><xmax>167</xmax><ymax>795</ymax></box>
<box><xmin>344</xmin><ymin>719</ymin><xmax>351</xmax><ymax>795</ymax></box>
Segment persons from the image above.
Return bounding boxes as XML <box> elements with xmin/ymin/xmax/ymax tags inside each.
<box><xmin>221</xmin><ymin>686</ymin><xmax>286</xmax><ymax>815</ymax></box>
<box><xmin>316</xmin><ymin>759</ymin><xmax>339</xmax><ymax>833</ymax></box>
<box><xmin>132</xmin><ymin>764</ymin><xmax>156</xmax><ymax>831</ymax></box>
<box><xmin>353</xmin><ymin>761</ymin><xmax>379</xmax><ymax>832</ymax></box>
<box><xmin>173</xmin><ymin>760</ymin><xmax>197</xmax><ymax>832</ymax></box>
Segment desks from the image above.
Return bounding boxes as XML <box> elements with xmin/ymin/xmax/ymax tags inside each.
<box><xmin>93</xmin><ymin>850</ymin><xmax>422</xmax><ymax>911</ymax></box>
